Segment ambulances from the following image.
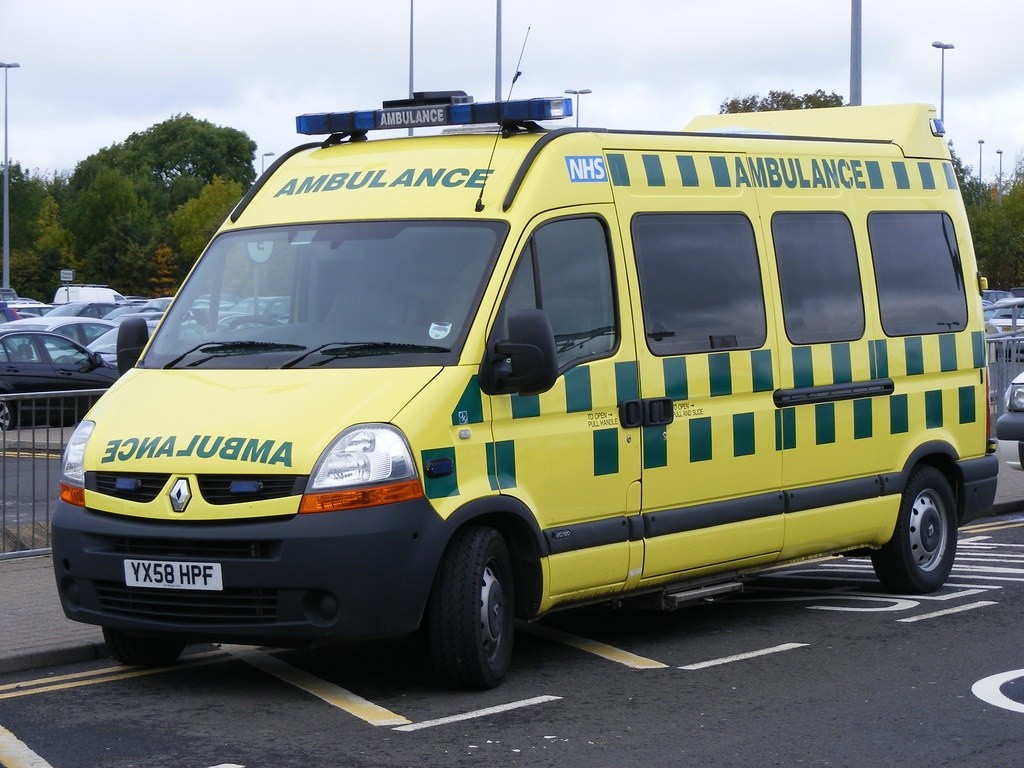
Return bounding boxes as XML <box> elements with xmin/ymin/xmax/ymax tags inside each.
<box><xmin>47</xmin><ymin>93</ymin><xmax>1000</xmax><ymax>694</ymax></box>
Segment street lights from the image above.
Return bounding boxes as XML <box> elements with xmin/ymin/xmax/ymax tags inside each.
<box><xmin>977</xmin><ymin>139</ymin><xmax>985</xmax><ymax>210</ymax></box>
<box><xmin>932</xmin><ymin>40</ymin><xmax>955</xmax><ymax>124</ymax></box>
<box><xmin>996</xmin><ymin>149</ymin><xmax>1004</xmax><ymax>206</ymax></box>
<box><xmin>0</xmin><ymin>62</ymin><xmax>20</xmax><ymax>291</ymax></box>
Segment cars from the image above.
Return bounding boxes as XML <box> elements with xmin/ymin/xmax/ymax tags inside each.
<box><xmin>0</xmin><ymin>330</ymin><xmax>122</xmax><ymax>433</ymax></box>
<box><xmin>0</xmin><ymin>283</ymin><xmax>292</xmax><ymax>367</ymax></box>
<box><xmin>982</xmin><ymin>290</ymin><xmax>1024</xmax><ymax>474</ymax></box>
